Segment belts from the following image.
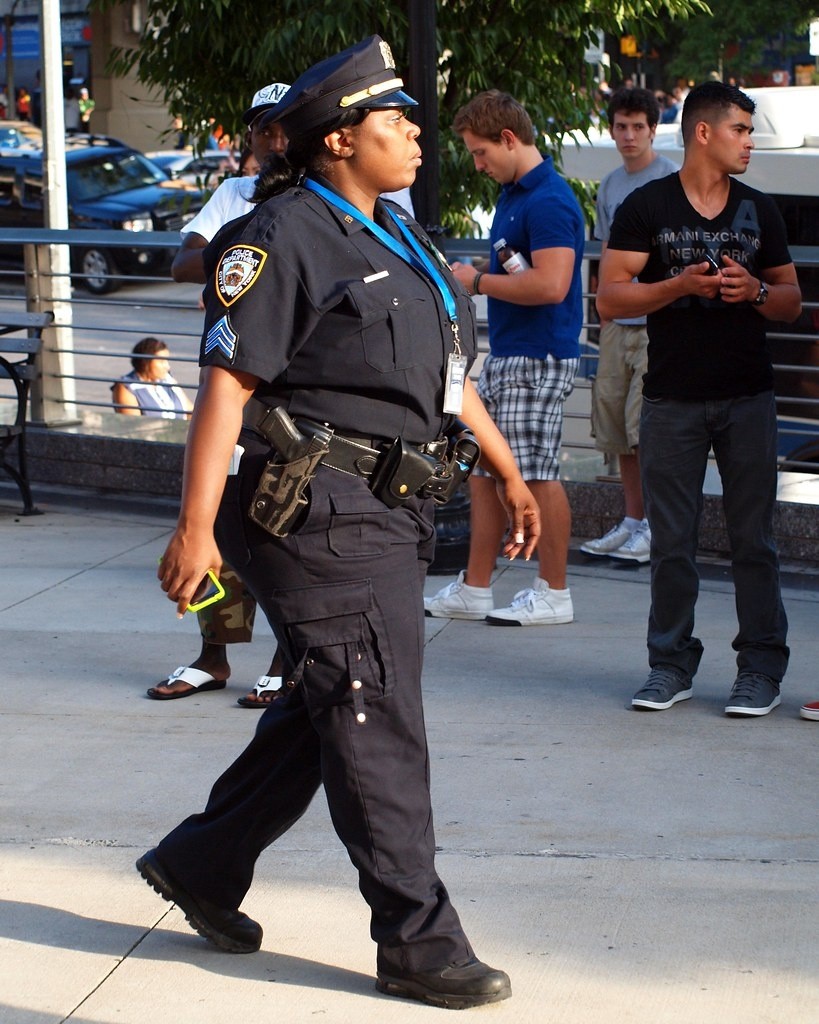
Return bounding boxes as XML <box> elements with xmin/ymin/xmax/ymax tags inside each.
<box><xmin>240</xmin><ymin>397</ymin><xmax>455</xmax><ymax>495</ymax></box>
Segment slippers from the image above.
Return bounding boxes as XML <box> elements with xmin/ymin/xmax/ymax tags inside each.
<box><xmin>146</xmin><ymin>667</ymin><xmax>227</xmax><ymax>699</ymax></box>
<box><xmin>238</xmin><ymin>675</ymin><xmax>283</xmax><ymax>708</ymax></box>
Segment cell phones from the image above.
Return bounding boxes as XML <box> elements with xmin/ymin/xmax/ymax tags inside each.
<box><xmin>158</xmin><ymin>555</ymin><xmax>225</xmax><ymax>612</ymax></box>
<box><xmin>700</xmin><ymin>254</ymin><xmax>719</xmax><ymax>276</ymax></box>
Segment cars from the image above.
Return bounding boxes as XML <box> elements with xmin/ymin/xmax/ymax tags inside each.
<box><xmin>141</xmin><ymin>148</ymin><xmax>241</xmax><ymax>178</ymax></box>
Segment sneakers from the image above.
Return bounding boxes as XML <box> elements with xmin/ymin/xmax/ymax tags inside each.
<box><xmin>581</xmin><ymin>523</ymin><xmax>634</xmax><ymax>555</ymax></box>
<box><xmin>486</xmin><ymin>577</ymin><xmax>574</xmax><ymax>626</ymax></box>
<box><xmin>631</xmin><ymin>665</ymin><xmax>695</xmax><ymax>710</ymax></box>
<box><xmin>135</xmin><ymin>850</ymin><xmax>263</xmax><ymax>953</ymax></box>
<box><xmin>607</xmin><ymin>522</ymin><xmax>654</xmax><ymax>562</ymax></box>
<box><xmin>424</xmin><ymin>570</ymin><xmax>493</xmax><ymax>620</ymax></box>
<box><xmin>376</xmin><ymin>958</ymin><xmax>511</xmax><ymax>1011</ymax></box>
<box><xmin>724</xmin><ymin>667</ymin><xmax>780</xmax><ymax>715</ymax></box>
<box><xmin>800</xmin><ymin>702</ymin><xmax>819</xmax><ymax>721</ymax></box>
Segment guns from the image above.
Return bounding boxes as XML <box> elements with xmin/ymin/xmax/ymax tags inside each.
<box><xmin>247</xmin><ymin>403</ymin><xmax>333</xmax><ymax>537</ymax></box>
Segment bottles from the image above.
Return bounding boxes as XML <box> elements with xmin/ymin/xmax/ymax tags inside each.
<box><xmin>492</xmin><ymin>238</ymin><xmax>531</xmax><ymax>275</ymax></box>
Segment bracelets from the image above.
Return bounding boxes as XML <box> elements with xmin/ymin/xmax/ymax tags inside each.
<box><xmin>473</xmin><ymin>272</ymin><xmax>483</xmax><ymax>295</ymax></box>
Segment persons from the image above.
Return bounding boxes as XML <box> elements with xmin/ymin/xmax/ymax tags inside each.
<box><xmin>421</xmin><ymin>89</ymin><xmax>585</xmax><ymax>626</ymax></box>
<box><xmin>137</xmin><ymin>36</ymin><xmax>542</xmax><ymax>1011</ymax></box>
<box><xmin>110</xmin><ymin>337</ymin><xmax>194</xmax><ymax>419</ymax></box>
<box><xmin>566</xmin><ymin>72</ymin><xmax>691</xmax><ymax>125</ymax></box>
<box><xmin>239</xmin><ymin>147</ymin><xmax>261</xmax><ymax>177</ymax></box>
<box><xmin>63</xmin><ymin>85</ymin><xmax>96</xmax><ymax>133</ymax></box>
<box><xmin>580</xmin><ymin>88</ymin><xmax>680</xmax><ymax>563</ymax></box>
<box><xmin>146</xmin><ymin>83</ymin><xmax>309</xmax><ymax>709</ymax></box>
<box><xmin>170</xmin><ymin>108</ymin><xmax>242</xmax><ymax>153</ymax></box>
<box><xmin>0</xmin><ymin>84</ymin><xmax>31</xmax><ymax>121</ymax></box>
<box><xmin>594</xmin><ymin>81</ymin><xmax>804</xmax><ymax>718</ymax></box>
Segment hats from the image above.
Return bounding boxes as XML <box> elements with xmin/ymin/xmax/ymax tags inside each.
<box><xmin>240</xmin><ymin>83</ymin><xmax>293</xmax><ymax>126</ymax></box>
<box><xmin>260</xmin><ymin>34</ymin><xmax>419</xmax><ymax>144</ymax></box>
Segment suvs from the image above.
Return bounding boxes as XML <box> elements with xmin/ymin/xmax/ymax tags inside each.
<box><xmin>0</xmin><ymin>118</ymin><xmax>216</xmax><ymax>296</ymax></box>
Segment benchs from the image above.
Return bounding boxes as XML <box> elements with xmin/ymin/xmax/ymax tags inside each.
<box><xmin>0</xmin><ymin>310</ymin><xmax>56</xmax><ymax>517</ymax></box>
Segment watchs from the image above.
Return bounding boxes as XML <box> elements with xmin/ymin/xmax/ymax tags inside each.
<box><xmin>752</xmin><ymin>282</ymin><xmax>768</xmax><ymax>306</ymax></box>
